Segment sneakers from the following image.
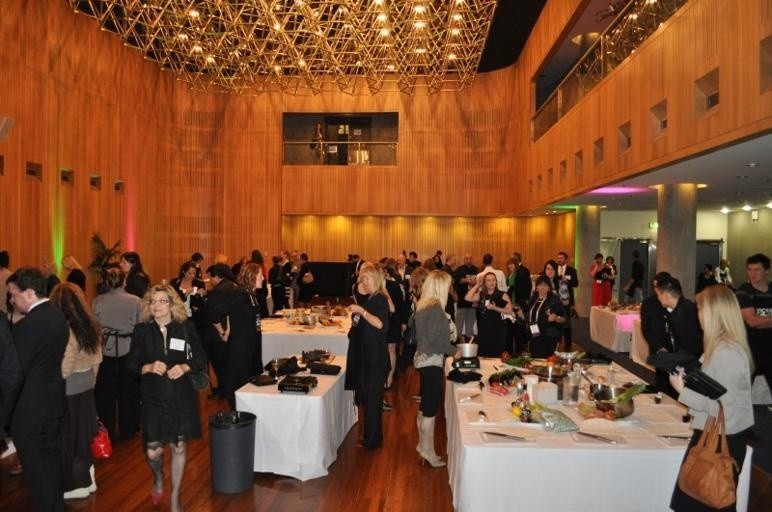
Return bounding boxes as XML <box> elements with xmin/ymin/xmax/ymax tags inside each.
<box><xmin>62</xmin><ymin>467</ymin><xmax>97</xmax><ymax>501</ymax></box>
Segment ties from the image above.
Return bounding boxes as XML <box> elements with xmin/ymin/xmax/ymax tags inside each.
<box><xmin>559</xmin><ymin>266</ymin><xmax>564</xmax><ymax>278</ymax></box>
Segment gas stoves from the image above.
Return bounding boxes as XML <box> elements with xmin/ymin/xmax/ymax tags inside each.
<box><xmin>275</xmin><ymin>376</ymin><xmax>318</xmax><ymax>394</ymax></box>
<box><xmin>453</xmin><ymin>357</ymin><xmax>480</xmax><ymax>368</ymax></box>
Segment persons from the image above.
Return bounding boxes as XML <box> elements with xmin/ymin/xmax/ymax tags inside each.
<box><xmin>653</xmin><ymin>276</ymin><xmax>703</xmax><ymax>396</ymax></box>
<box><xmin>621</xmin><ymin>249</ymin><xmax>643</xmax><ymax>304</ymax></box>
<box><xmin>604</xmin><ymin>256</ymin><xmax>617</xmax><ymax>289</ymax></box>
<box><xmin>668</xmin><ymin>285</ymin><xmax>754</xmax><ymax>511</ymax></box>
<box><xmin>735</xmin><ymin>254</ymin><xmax>771</xmax><ymax>409</ymax></box>
<box><xmin>697</xmin><ymin>263</ymin><xmax>717</xmax><ymax>294</ymax></box>
<box><xmin>588</xmin><ymin>254</ymin><xmax>612</xmax><ymax>305</ymax></box>
<box><xmin>640</xmin><ymin>273</ymin><xmax>672</xmax><ymax>395</ymax></box>
<box><xmin>714</xmin><ymin>259</ymin><xmax>732</xmax><ymax>284</ymax></box>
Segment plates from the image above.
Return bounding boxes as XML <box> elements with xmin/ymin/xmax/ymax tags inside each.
<box><xmin>463</xmin><ymin>409</ymin><xmax>512</xmax><ymax>426</ymax></box>
<box><xmin>480</xmin><ymin>429</ymin><xmax>696</xmax><ymax>447</ymax></box>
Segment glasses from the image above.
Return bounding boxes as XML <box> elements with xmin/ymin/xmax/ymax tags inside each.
<box><xmin>148</xmin><ymin>299</ymin><xmax>170</xmax><ymax>305</ymax></box>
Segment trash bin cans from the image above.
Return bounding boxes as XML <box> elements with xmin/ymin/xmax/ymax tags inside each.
<box><xmin>209</xmin><ymin>411</ymin><xmax>256</xmax><ymax>494</ymax></box>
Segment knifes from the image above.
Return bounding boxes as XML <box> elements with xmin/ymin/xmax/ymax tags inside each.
<box><xmin>458</xmin><ymin>391</ymin><xmax>480</xmax><ymax>403</ymax></box>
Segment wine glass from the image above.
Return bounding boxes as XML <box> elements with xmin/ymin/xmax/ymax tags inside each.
<box><xmin>323</xmin><ymin>345</ymin><xmax>333</xmax><ymax>364</ymax></box>
<box><xmin>271</xmin><ymin>353</ymin><xmax>283</xmax><ymax>381</ymax></box>
<box><xmin>282</xmin><ymin>307</ymin><xmax>333</xmax><ymax>327</ymax></box>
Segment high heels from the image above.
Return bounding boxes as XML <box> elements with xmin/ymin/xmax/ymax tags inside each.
<box><xmin>416</xmin><ymin>444</ymin><xmax>447</xmax><ymax>469</ymax></box>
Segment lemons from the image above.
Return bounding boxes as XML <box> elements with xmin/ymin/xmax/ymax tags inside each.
<box><xmin>512</xmin><ymin>406</ymin><xmax>523</xmax><ymax>416</ymax></box>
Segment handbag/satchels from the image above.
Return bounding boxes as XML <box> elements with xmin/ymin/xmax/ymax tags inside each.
<box><xmin>91</xmin><ymin>420</ymin><xmax>113</xmax><ymax>460</ymax></box>
<box><xmin>182</xmin><ymin>321</ymin><xmax>211</xmax><ymax>391</ymax></box>
<box><xmin>676</xmin><ymin>400</ymin><xmax>740</xmax><ymax>509</ymax></box>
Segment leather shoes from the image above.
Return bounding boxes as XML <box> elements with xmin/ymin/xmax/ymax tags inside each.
<box><xmin>354</xmin><ymin>433</ymin><xmax>377</xmax><ymax>449</ymax></box>
<box><xmin>150</xmin><ymin>477</ymin><xmax>165</xmax><ymax>506</ymax></box>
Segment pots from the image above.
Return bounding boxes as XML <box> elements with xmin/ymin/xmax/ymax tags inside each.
<box><xmin>482</xmin><ymin>350</ymin><xmax>635</xmax><ymax>423</ymax></box>
<box><xmin>456</xmin><ymin>335</ymin><xmax>479</xmax><ymax>358</ymax></box>
